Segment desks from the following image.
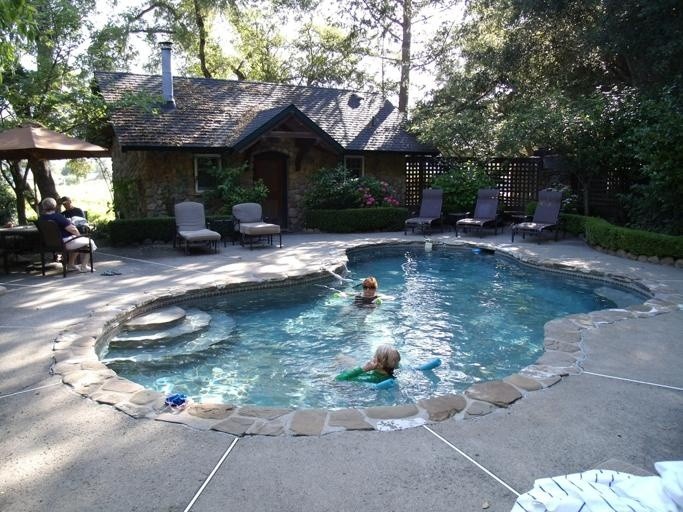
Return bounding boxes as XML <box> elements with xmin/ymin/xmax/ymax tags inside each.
<box><xmin>0</xmin><ymin>224</ymin><xmax>61</xmax><ymax>276</ymax></box>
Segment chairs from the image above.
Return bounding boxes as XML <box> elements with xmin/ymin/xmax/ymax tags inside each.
<box><xmin>173</xmin><ymin>200</ymin><xmax>283</xmax><ymax>257</ymax></box>
<box><xmin>33</xmin><ymin>210</ymin><xmax>94</xmax><ymax>278</ymax></box>
<box><xmin>403</xmin><ymin>188</ymin><xmax>566</xmax><ymax>245</ymax></box>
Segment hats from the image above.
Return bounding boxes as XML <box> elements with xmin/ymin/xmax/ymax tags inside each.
<box><xmin>60</xmin><ymin>196</ymin><xmax>72</xmax><ymax>202</ymax></box>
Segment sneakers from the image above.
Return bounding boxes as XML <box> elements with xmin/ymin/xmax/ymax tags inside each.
<box><xmin>67</xmin><ymin>264</ymin><xmax>79</xmax><ymax>271</ymax></box>
<box><xmin>80</xmin><ymin>264</ymin><xmax>97</xmax><ymax>273</ymax></box>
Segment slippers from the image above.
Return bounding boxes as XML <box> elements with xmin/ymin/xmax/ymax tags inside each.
<box><xmin>100</xmin><ymin>269</ymin><xmax>121</xmax><ymax>276</ymax></box>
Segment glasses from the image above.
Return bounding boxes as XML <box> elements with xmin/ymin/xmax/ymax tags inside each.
<box><xmin>62</xmin><ymin>202</ymin><xmax>71</xmax><ymax>205</ymax></box>
<box><xmin>363</xmin><ymin>285</ymin><xmax>376</xmax><ymax>290</ymax></box>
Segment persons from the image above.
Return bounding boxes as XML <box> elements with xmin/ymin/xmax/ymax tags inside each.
<box><xmin>38</xmin><ymin>196</ymin><xmax>97</xmax><ymax>272</ymax></box>
<box><xmin>355</xmin><ymin>276</ymin><xmax>381</xmax><ymax>307</ymax></box>
<box><xmin>335</xmin><ymin>343</ymin><xmax>401</xmax><ymax>383</ymax></box>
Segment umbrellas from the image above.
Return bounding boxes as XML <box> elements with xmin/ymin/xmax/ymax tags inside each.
<box><xmin>0</xmin><ymin>126</ymin><xmax>109</xmax><ymax>214</ymax></box>
